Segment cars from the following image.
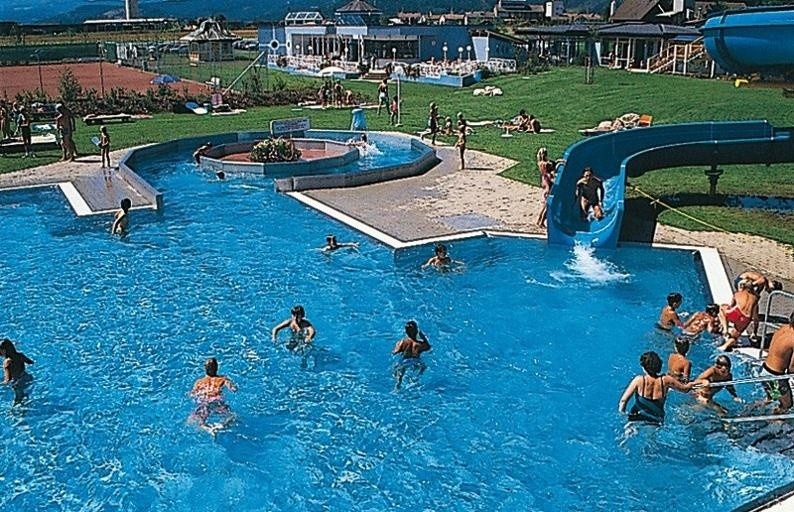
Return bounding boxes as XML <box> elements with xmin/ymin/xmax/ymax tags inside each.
<box><xmin>233</xmin><ymin>37</ymin><xmax>259</xmax><ymax>52</ymax></box>
<box><xmin>135</xmin><ymin>40</ymin><xmax>190</xmax><ymax>60</ymax></box>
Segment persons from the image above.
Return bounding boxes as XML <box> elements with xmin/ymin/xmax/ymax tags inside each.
<box><xmin>571</xmin><ymin>165</ymin><xmax>606</xmax><ymax>223</ymax></box>
<box><xmin>316</xmin><ymin>232</ymin><xmax>359</xmax><ymax>256</ymax></box>
<box><xmin>744</xmin><ymin>309</ymin><xmax>793</xmax><ymax>431</ymax></box>
<box><xmin>275</xmin><ymin>53</ymin><xmax>421</xmax><ymax>82</ymax></box>
<box><xmin>653</xmin><ymin>292</ymin><xmax>684</xmax><ymax>337</ymax></box>
<box><xmin>413</xmin><ymin>101</ymin><xmax>476</xmax><ymax>172</ymax></box>
<box><xmin>679</xmin><ymin>302</ymin><xmax>721</xmax><ymax>345</ymax></box>
<box><xmin>419</xmin><ymin>243</ymin><xmax>465</xmax><ymax>277</ymax></box>
<box><xmin>692</xmin><ymin>354</ymin><xmax>745</xmax><ymax>416</ymax></box>
<box><xmin>716</xmin><ymin>282</ymin><xmax>758</xmax><ymax>354</ymax></box>
<box><xmin>186</xmin><ymin>355</ymin><xmax>239</xmax><ymax>439</ymax></box>
<box><xmin>108</xmin><ymin>197</ymin><xmax>131</xmax><ymax>240</ymax></box>
<box><xmin>313</xmin><ymin>82</ymin><xmax>403</xmax><ymax>131</ymax></box>
<box><xmin>734</xmin><ymin>270</ymin><xmax>783</xmax><ymax>300</ymax></box>
<box><xmin>535</xmin><ymin>146</ymin><xmax>567</xmax><ymax>205</ymax></box>
<box><xmin>0</xmin><ymin>98</ymin><xmax>79</xmax><ymax>163</ymax></box>
<box><xmin>0</xmin><ymin>339</ymin><xmax>32</xmax><ymax>409</ymax></box>
<box><xmin>665</xmin><ymin>335</ymin><xmax>692</xmax><ymax>383</ymax></box>
<box><xmin>536</xmin><ymin>160</ymin><xmax>556</xmax><ymax>230</ymax></box>
<box><xmin>344</xmin><ymin>134</ymin><xmax>369</xmax><ymax>157</ymax></box>
<box><xmin>498</xmin><ymin>109</ymin><xmax>544</xmax><ymax>138</ymax></box>
<box><xmin>95</xmin><ymin>125</ymin><xmax>110</xmax><ymax>169</ymax></box>
<box><xmin>615</xmin><ymin>350</ymin><xmax>703</xmax><ymax>447</ymax></box>
<box><xmin>390</xmin><ymin>321</ymin><xmax>431</xmax><ymax>391</ymax></box>
<box><xmin>272</xmin><ymin>305</ymin><xmax>315</xmax><ymax>350</ymax></box>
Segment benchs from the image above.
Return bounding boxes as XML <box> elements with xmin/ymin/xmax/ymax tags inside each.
<box><xmin>84</xmin><ymin>113</ymin><xmax>130</xmax><ymax>126</ymax></box>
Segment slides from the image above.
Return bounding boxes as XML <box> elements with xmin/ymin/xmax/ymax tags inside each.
<box><xmin>547</xmin><ymin>5</ymin><xmax>794</xmax><ymax>250</ymax></box>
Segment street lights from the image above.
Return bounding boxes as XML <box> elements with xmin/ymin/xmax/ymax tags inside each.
<box><xmin>296</xmin><ymin>44</ymin><xmax>300</xmax><ymax>56</ymax></box>
<box><xmin>391</xmin><ymin>47</ymin><xmax>396</xmax><ymax>62</ymax></box>
<box><xmin>308</xmin><ymin>45</ymin><xmax>312</xmax><ymax>56</ymax></box>
<box><xmin>95</xmin><ymin>39</ymin><xmax>106</xmax><ymax>98</ymax></box>
<box><xmin>485</xmin><ymin>46</ymin><xmax>490</xmax><ymax>61</ymax></box>
<box><xmin>442</xmin><ymin>45</ymin><xmax>448</xmax><ymax>62</ymax></box>
<box><xmin>466</xmin><ymin>45</ymin><xmax>472</xmax><ymax>61</ymax></box>
<box><xmin>344</xmin><ymin>46</ymin><xmax>349</xmax><ymax>61</ymax></box>
<box><xmin>29</xmin><ymin>54</ymin><xmax>46</xmax><ymax>99</ymax></box>
<box><xmin>458</xmin><ymin>46</ymin><xmax>464</xmax><ymax>61</ymax></box>
